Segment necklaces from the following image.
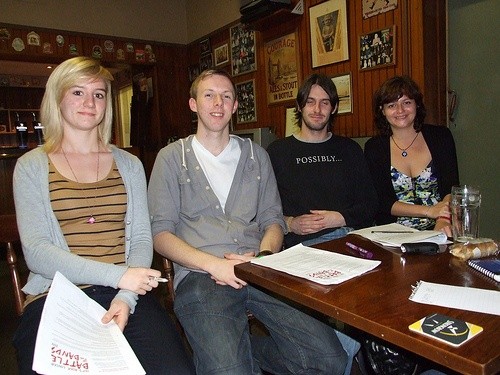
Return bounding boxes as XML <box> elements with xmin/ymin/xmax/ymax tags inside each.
<box><xmin>391</xmin><ymin>133</ymin><xmax>419</xmax><ymax>157</ymax></box>
<box><xmin>60</xmin><ymin>137</ymin><xmax>100</xmax><ymax>223</ymax></box>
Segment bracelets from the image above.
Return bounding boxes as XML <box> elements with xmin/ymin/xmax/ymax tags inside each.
<box><xmin>255</xmin><ymin>250</ymin><xmax>273</xmax><ymax>257</ymax></box>
<box><xmin>437</xmin><ymin>216</ymin><xmax>452</xmax><ymax>224</ymax></box>
<box><xmin>425</xmin><ymin>204</ymin><xmax>432</xmax><ymax>217</ymax></box>
<box><xmin>286</xmin><ymin>216</ymin><xmax>293</xmax><ymax>232</ymax></box>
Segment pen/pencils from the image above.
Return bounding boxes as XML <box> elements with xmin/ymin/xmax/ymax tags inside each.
<box><xmin>371</xmin><ymin>230</ymin><xmax>414</xmax><ymax>234</ymax></box>
<box><xmin>346</xmin><ymin>242</ymin><xmax>373</xmax><ymax>258</ymax></box>
<box><xmin>148</xmin><ymin>276</ymin><xmax>168</xmax><ymax>282</ymax></box>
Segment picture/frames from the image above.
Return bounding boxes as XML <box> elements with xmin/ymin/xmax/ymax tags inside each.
<box><xmin>329</xmin><ymin>71</ymin><xmax>354</xmax><ymax>116</ymax></box>
<box><xmin>188</xmin><ymin>22</ymin><xmax>257</xmax><ymax>123</ymax></box>
<box><xmin>263</xmin><ymin>26</ymin><xmax>303</xmax><ymax>107</ymax></box>
<box><xmin>357</xmin><ymin>24</ymin><xmax>397</xmax><ymax>71</ymax></box>
<box><xmin>308</xmin><ymin>0</ymin><xmax>350</xmax><ymax>69</ymax></box>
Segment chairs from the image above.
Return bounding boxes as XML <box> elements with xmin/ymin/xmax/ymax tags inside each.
<box><xmin>0</xmin><ymin>214</ymin><xmax>28</xmax><ymax>321</ymax></box>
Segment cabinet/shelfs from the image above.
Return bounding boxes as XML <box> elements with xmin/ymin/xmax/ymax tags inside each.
<box><xmin>0</xmin><ymin>107</ymin><xmax>40</xmax><ymax>149</ymax></box>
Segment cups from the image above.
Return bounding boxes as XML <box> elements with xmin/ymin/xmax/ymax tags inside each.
<box><xmin>450</xmin><ymin>186</ymin><xmax>481</xmax><ymax>243</ymax></box>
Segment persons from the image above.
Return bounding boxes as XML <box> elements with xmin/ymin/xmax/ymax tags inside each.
<box><xmin>12</xmin><ymin>58</ymin><xmax>198</xmax><ymax>375</ymax></box>
<box><xmin>237</xmin><ymin>82</ymin><xmax>256</xmax><ymax>121</ymax></box>
<box><xmin>361</xmin><ymin>33</ymin><xmax>393</xmax><ymax>69</ymax></box>
<box><xmin>147</xmin><ymin>68</ymin><xmax>349</xmax><ymax>375</ymax></box>
<box><xmin>232</xmin><ymin>23</ymin><xmax>254</xmax><ymax>73</ymax></box>
<box><xmin>364</xmin><ymin>75</ymin><xmax>465</xmax><ymax>238</ymax></box>
<box><xmin>321</xmin><ymin>14</ymin><xmax>334</xmax><ymax>48</ymax></box>
<box><xmin>266</xmin><ymin>72</ymin><xmax>370</xmax><ymax>375</ymax></box>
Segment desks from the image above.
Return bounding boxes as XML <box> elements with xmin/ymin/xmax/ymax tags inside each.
<box><xmin>233</xmin><ymin>222</ymin><xmax>500</xmax><ymax>375</ymax></box>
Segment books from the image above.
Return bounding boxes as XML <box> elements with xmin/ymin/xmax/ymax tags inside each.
<box><xmin>409</xmin><ymin>313</ymin><xmax>483</xmax><ymax>347</ymax></box>
<box><xmin>468</xmin><ymin>258</ymin><xmax>500</xmax><ymax>282</ymax></box>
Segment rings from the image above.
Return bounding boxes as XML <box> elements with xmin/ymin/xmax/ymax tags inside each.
<box><xmin>125</xmin><ymin>321</ymin><xmax>128</xmax><ymax>326</ymax></box>
<box><xmin>147</xmin><ymin>278</ymin><xmax>151</xmax><ymax>285</ymax></box>
